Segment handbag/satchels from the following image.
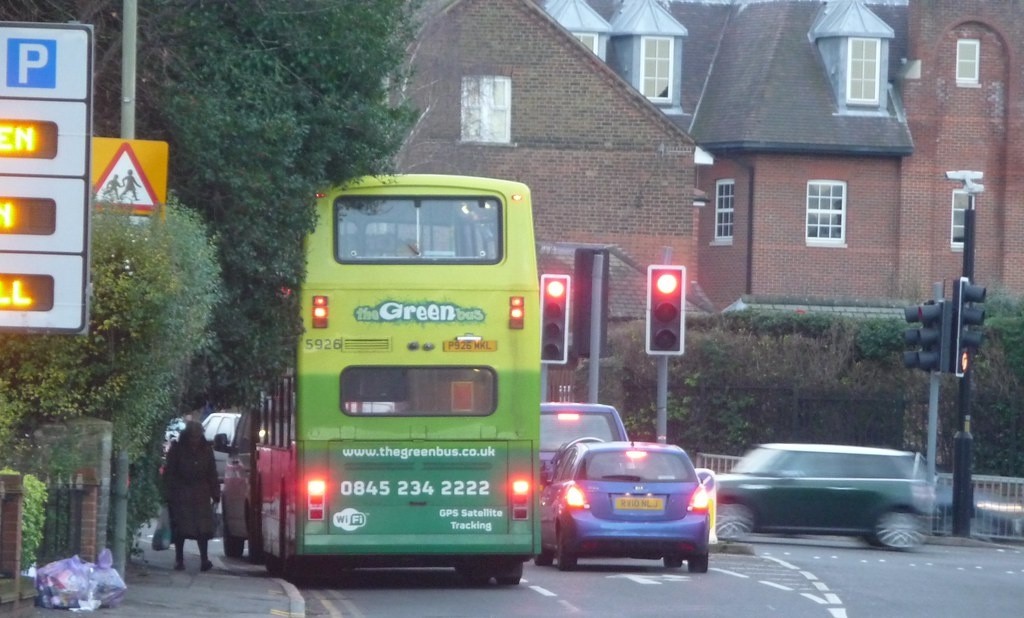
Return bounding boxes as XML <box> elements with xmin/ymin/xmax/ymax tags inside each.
<box><xmin>151</xmin><ymin>504</ymin><xmax>172</xmax><ymax>550</ymax></box>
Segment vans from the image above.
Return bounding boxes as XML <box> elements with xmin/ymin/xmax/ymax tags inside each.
<box><xmin>211</xmin><ymin>400</ymin><xmax>254</xmax><ymax>558</ymax></box>
<box><xmin>198</xmin><ymin>413</ymin><xmax>242</xmax><ymax>481</ymax></box>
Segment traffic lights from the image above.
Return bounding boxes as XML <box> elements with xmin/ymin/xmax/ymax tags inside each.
<box><xmin>643</xmin><ymin>264</ymin><xmax>686</xmax><ymax>357</ymax></box>
<box><xmin>900</xmin><ymin>298</ymin><xmax>936</xmax><ymax>372</ymax></box>
<box><xmin>949</xmin><ymin>276</ymin><xmax>987</xmax><ymax>379</ymax></box>
<box><xmin>915</xmin><ymin>300</ymin><xmax>951</xmax><ymax>375</ymax></box>
<box><xmin>540</xmin><ymin>272</ymin><xmax>571</xmax><ymax>366</ymax></box>
<box><xmin>0</xmin><ymin>22</ymin><xmax>92</xmax><ymax>338</ymax></box>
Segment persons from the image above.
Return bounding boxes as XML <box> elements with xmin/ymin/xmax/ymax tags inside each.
<box><xmin>156</xmin><ymin>420</ymin><xmax>222</xmax><ymax>570</ymax></box>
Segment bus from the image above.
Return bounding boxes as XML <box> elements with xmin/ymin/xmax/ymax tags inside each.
<box><xmin>251</xmin><ymin>171</ymin><xmax>542</xmax><ymax>587</ymax></box>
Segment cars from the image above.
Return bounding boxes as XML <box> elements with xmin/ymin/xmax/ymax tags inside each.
<box><xmin>534</xmin><ymin>441</ymin><xmax>710</xmax><ymax>574</ymax></box>
<box><xmin>157</xmin><ymin>417</ymin><xmax>188</xmax><ymax>472</ymax></box>
<box><xmin>933</xmin><ymin>483</ymin><xmax>1024</xmax><ymax>544</ymax></box>
<box><xmin>714</xmin><ymin>441</ymin><xmax>932</xmax><ymax>550</ymax></box>
<box><xmin>538</xmin><ymin>400</ymin><xmax>628</xmax><ymax>488</ymax></box>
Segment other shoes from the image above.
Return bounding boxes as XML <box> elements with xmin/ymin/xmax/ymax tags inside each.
<box><xmin>175</xmin><ymin>559</ymin><xmax>185</xmax><ymax>570</ymax></box>
<box><xmin>201</xmin><ymin>559</ymin><xmax>213</xmax><ymax>571</ymax></box>
